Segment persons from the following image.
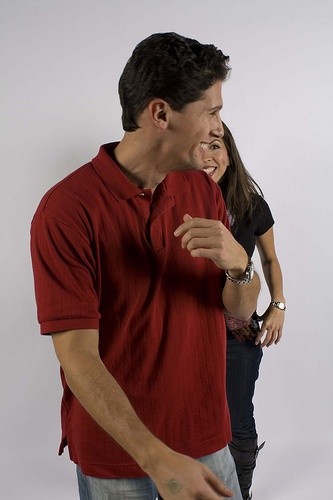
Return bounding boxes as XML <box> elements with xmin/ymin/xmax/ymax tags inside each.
<box><xmin>31</xmin><ymin>32</ymin><xmax>261</xmax><ymax>500</ymax></box>
<box><xmin>158</xmin><ymin>120</ymin><xmax>286</xmax><ymax>500</ymax></box>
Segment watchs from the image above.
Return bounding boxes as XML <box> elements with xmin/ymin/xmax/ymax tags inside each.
<box><xmin>225</xmin><ymin>255</ymin><xmax>254</xmax><ymax>284</ymax></box>
<box><xmin>269</xmin><ymin>301</ymin><xmax>286</xmax><ymax>311</ymax></box>
